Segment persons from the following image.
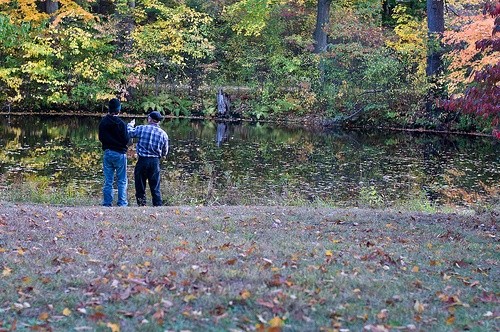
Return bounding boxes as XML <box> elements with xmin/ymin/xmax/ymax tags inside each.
<box><xmin>98</xmin><ymin>98</ymin><xmax>136</xmax><ymax>207</ymax></box>
<box><xmin>125</xmin><ymin>111</ymin><xmax>169</xmax><ymax>207</ymax></box>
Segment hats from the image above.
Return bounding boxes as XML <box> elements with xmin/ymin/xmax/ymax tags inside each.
<box><xmin>148</xmin><ymin>111</ymin><xmax>164</xmax><ymax>120</ymax></box>
<box><xmin>108</xmin><ymin>98</ymin><xmax>121</xmax><ymax>111</ymax></box>
